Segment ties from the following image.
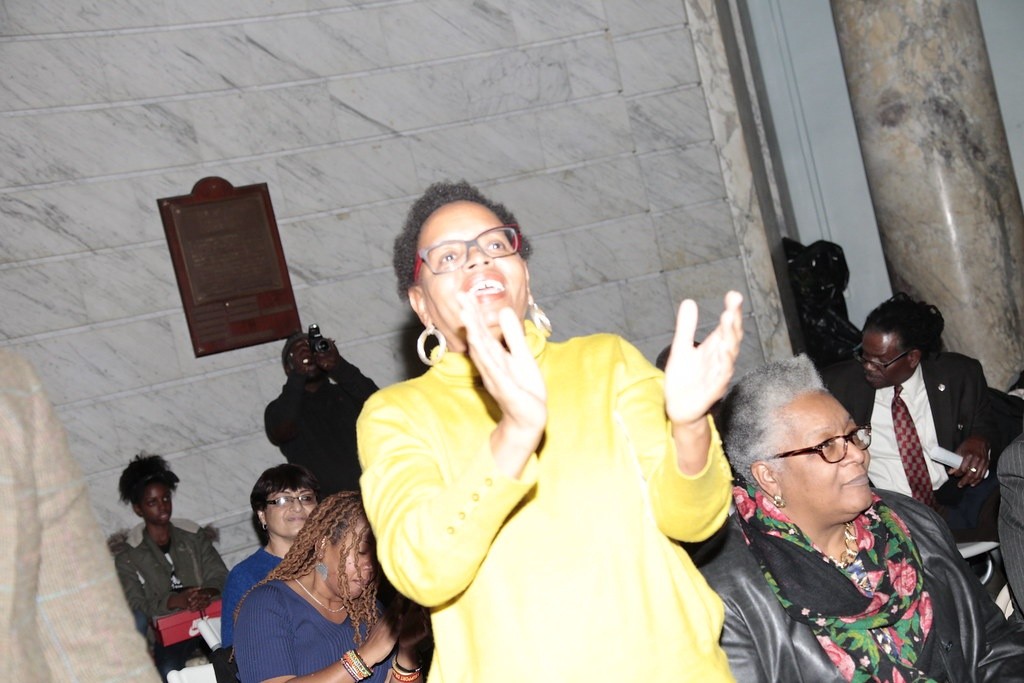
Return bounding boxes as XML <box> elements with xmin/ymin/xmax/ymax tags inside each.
<box><xmin>892</xmin><ymin>385</ymin><xmax>936</xmax><ymax>511</ymax></box>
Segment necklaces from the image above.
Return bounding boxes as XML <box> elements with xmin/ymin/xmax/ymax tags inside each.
<box><xmin>293</xmin><ymin>579</ymin><xmax>345</xmax><ymax>613</ymax></box>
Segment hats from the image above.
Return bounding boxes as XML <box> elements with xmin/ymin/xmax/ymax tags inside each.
<box><xmin>282</xmin><ymin>332</ymin><xmax>308</xmax><ymax>361</ymax></box>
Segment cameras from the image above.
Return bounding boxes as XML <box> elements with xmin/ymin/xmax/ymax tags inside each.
<box><xmin>306</xmin><ymin>324</ymin><xmax>331</xmax><ymax>356</ymax></box>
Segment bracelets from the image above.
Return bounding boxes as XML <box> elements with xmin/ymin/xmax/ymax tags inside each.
<box><xmin>391</xmin><ymin>653</ymin><xmax>423</xmax><ymax>683</ymax></box>
<box><xmin>340</xmin><ymin>648</ymin><xmax>373</xmax><ymax>683</ymax></box>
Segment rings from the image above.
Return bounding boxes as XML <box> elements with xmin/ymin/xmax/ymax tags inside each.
<box><xmin>323</xmin><ymin>363</ymin><xmax>328</xmax><ymax>368</ymax></box>
<box><xmin>970</xmin><ymin>466</ymin><xmax>978</xmax><ymax>473</ymax></box>
<box><xmin>303</xmin><ymin>359</ymin><xmax>309</xmax><ymax>364</ymax></box>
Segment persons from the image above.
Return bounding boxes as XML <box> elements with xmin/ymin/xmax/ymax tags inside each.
<box><xmin>997</xmin><ymin>434</ymin><xmax>1024</xmax><ymax>612</ymax></box>
<box><xmin>830</xmin><ymin>301</ymin><xmax>1001</xmax><ymax>546</ymax></box>
<box><xmin>0</xmin><ymin>346</ymin><xmax>165</xmax><ymax>683</ymax></box>
<box><xmin>650</xmin><ymin>337</ymin><xmax>1024</xmax><ymax>683</ymax></box>
<box><xmin>354</xmin><ymin>181</ymin><xmax>744</xmax><ymax>683</ymax></box>
<box><xmin>262</xmin><ymin>335</ymin><xmax>383</xmax><ymax>504</ymax></box>
<box><xmin>218</xmin><ymin>463</ymin><xmax>435</xmax><ymax>683</ymax></box>
<box><xmin>109</xmin><ymin>455</ymin><xmax>240</xmax><ymax>683</ymax></box>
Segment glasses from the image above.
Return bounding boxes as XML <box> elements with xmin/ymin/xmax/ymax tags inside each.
<box><xmin>853</xmin><ymin>342</ymin><xmax>911</xmax><ymax>370</ymax></box>
<box><xmin>414</xmin><ymin>224</ymin><xmax>522</xmax><ymax>280</ymax></box>
<box><xmin>773</xmin><ymin>424</ymin><xmax>871</xmax><ymax>464</ymax></box>
<box><xmin>266</xmin><ymin>494</ymin><xmax>316</xmax><ymax>508</ymax></box>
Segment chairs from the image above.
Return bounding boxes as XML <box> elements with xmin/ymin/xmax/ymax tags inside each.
<box><xmin>957</xmin><ymin>540</ymin><xmax>1024</xmax><ymax>624</ymax></box>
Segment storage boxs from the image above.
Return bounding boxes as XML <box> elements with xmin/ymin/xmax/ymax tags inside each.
<box><xmin>157</xmin><ymin>600</ymin><xmax>222</xmax><ymax>648</ymax></box>
